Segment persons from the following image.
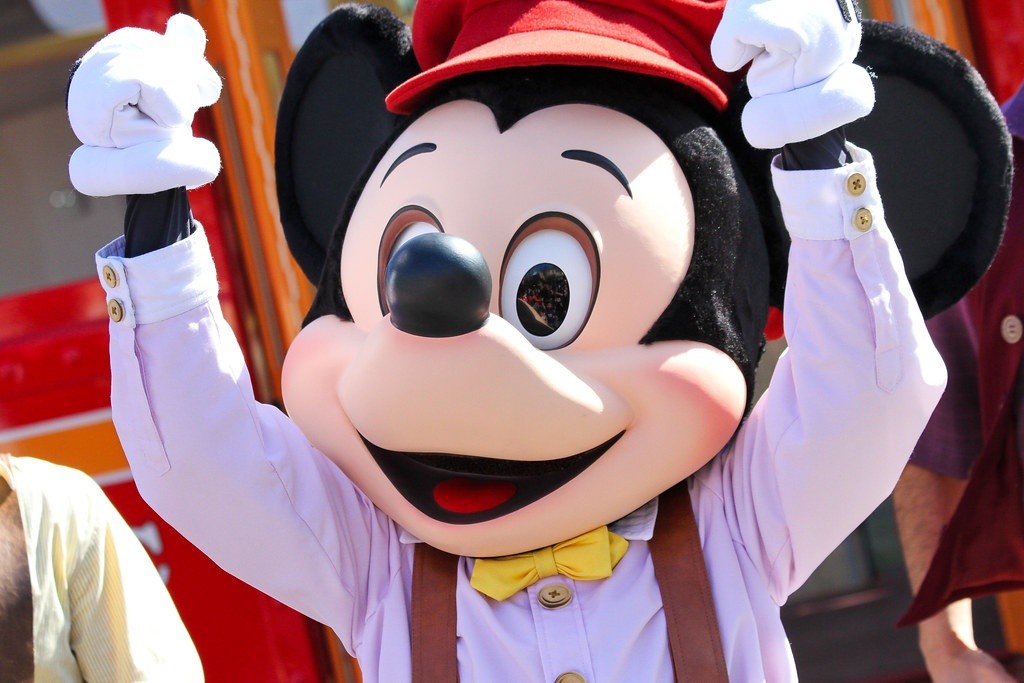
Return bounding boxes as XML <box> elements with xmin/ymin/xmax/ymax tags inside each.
<box><xmin>0</xmin><ymin>454</ymin><xmax>207</xmax><ymax>683</ymax></box>
<box><xmin>896</xmin><ymin>87</ymin><xmax>1023</xmax><ymax>683</ymax></box>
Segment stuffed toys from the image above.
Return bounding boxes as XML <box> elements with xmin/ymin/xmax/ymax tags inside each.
<box><xmin>67</xmin><ymin>0</ymin><xmax>1014</xmax><ymax>683</ymax></box>
